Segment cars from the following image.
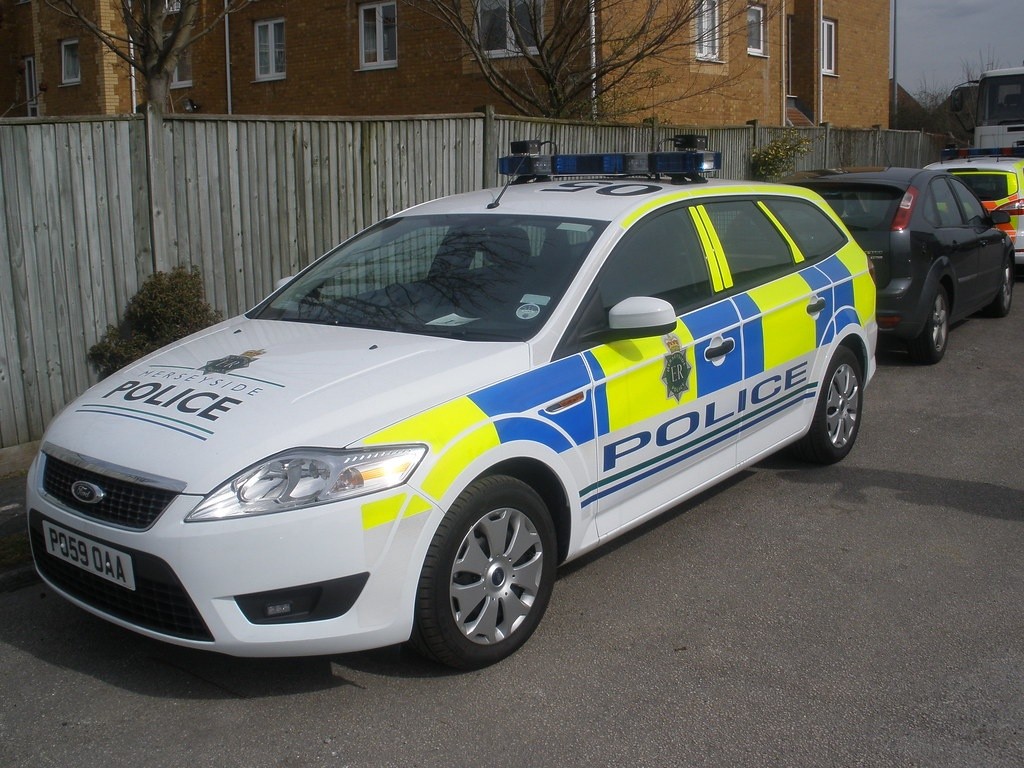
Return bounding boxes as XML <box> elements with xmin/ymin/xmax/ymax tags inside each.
<box><xmin>774</xmin><ymin>151</ymin><xmax>1024</xmax><ymax>365</ymax></box>
<box><xmin>26</xmin><ymin>112</ymin><xmax>878</xmax><ymax>670</ymax></box>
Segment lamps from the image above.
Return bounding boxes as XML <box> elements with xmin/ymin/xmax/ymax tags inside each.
<box><xmin>180</xmin><ymin>98</ymin><xmax>200</xmax><ymax>112</ymax></box>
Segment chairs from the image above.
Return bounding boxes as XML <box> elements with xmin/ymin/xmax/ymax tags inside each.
<box><xmin>1004</xmin><ymin>93</ymin><xmax>1024</xmax><ymax>110</ymax></box>
<box><xmin>869</xmin><ymin>194</ymin><xmax>891</xmax><ymax>217</ymax></box>
<box><xmin>823</xmin><ymin>194</ymin><xmax>845</xmax><ymax>217</ymax></box>
<box><xmin>472</xmin><ymin>226</ymin><xmax>542</xmax><ymax>301</ymax></box>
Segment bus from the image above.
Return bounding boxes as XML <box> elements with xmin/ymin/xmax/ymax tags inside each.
<box><xmin>951</xmin><ymin>67</ymin><xmax>1024</xmax><ymax>158</ymax></box>
